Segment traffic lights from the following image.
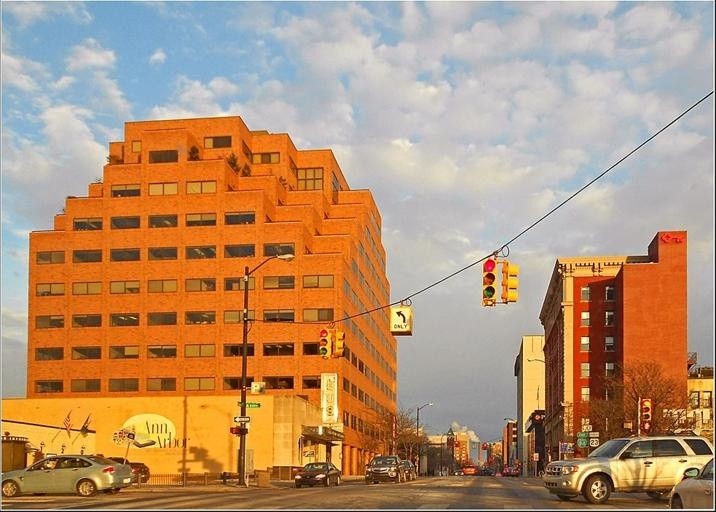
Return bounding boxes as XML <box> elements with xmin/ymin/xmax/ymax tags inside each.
<box><xmin>231</xmin><ymin>428</ymin><xmax>248</xmax><ymax>434</ymax></box>
<box><xmin>641</xmin><ymin>398</ymin><xmax>652</xmax><ymax>422</ymax></box>
<box><xmin>482</xmin><ymin>256</ymin><xmax>520</xmax><ymax>308</ymax></box>
<box><xmin>319</xmin><ymin>328</ymin><xmax>345</xmax><ymax>359</ymax></box>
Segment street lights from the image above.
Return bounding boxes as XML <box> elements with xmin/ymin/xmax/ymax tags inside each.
<box><xmin>416</xmin><ymin>401</ymin><xmax>434</xmax><ymax>444</ymax></box>
<box><xmin>239</xmin><ymin>253</ymin><xmax>295</xmax><ymax>486</ymax></box>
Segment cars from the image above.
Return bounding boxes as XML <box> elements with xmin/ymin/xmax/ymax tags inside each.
<box><xmin>503</xmin><ymin>466</ymin><xmax>520</xmax><ymax>477</ymax></box>
<box><xmin>544</xmin><ymin>436</ymin><xmax>713</xmax><ymax>508</ymax></box>
<box><xmin>295</xmin><ymin>462</ymin><xmax>342</xmax><ymax>488</ymax></box>
<box><xmin>0</xmin><ymin>455</ymin><xmax>150</xmax><ymax>497</ymax></box>
<box><xmin>366</xmin><ymin>456</ymin><xmax>416</xmax><ymax>485</ymax></box>
<box><xmin>454</xmin><ymin>465</ymin><xmax>478</xmax><ymax>476</ymax></box>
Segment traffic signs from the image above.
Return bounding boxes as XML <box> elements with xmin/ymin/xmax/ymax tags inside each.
<box><xmin>234</xmin><ymin>403</ymin><xmax>260</xmax><ymax>422</ymax></box>
